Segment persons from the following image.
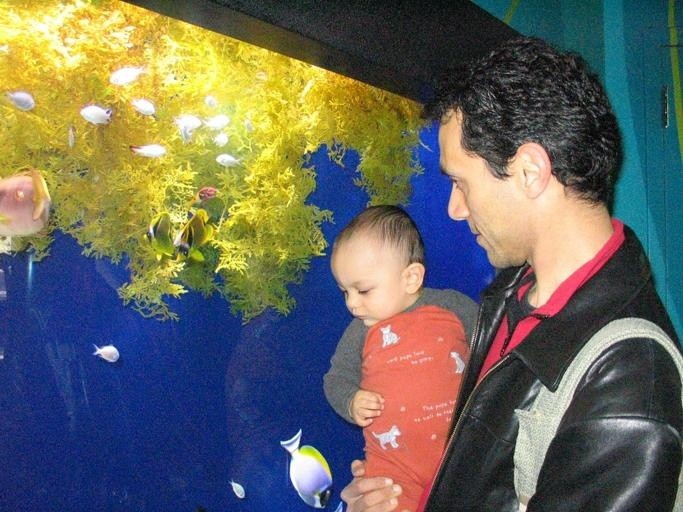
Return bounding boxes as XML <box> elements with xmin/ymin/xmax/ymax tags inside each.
<box><xmin>338</xmin><ymin>32</ymin><xmax>682</xmax><ymax>511</ymax></box>
<box><xmin>319</xmin><ymin>200</ymin><xmax>482</xmax><ymax>512</ymax></box>
